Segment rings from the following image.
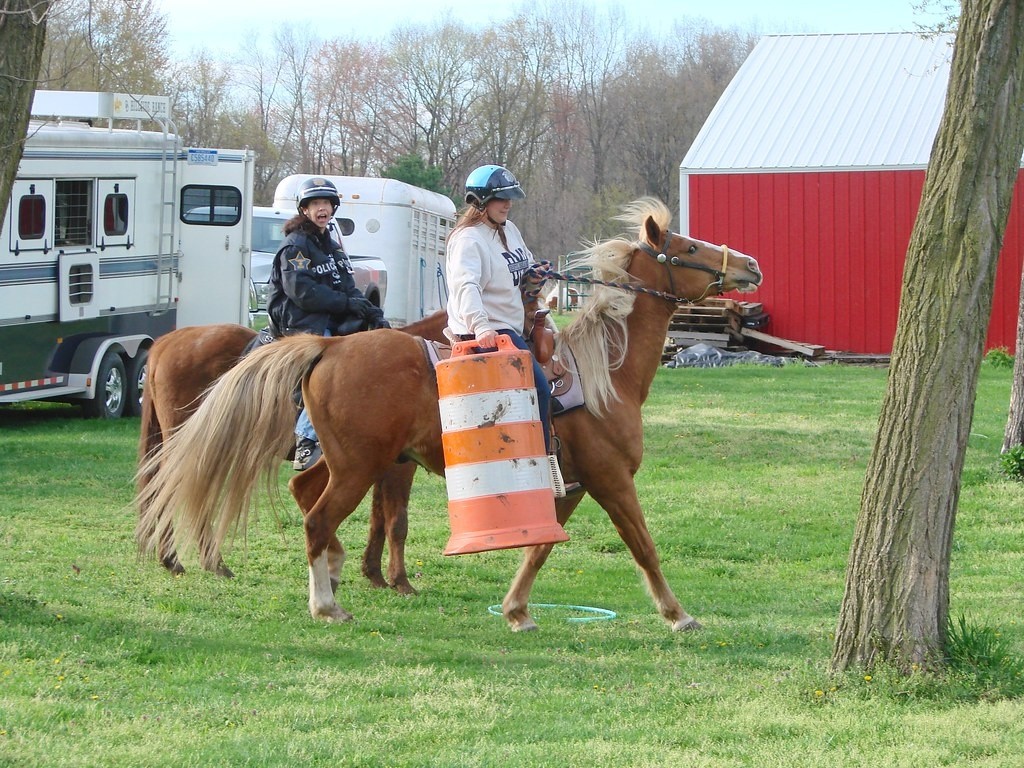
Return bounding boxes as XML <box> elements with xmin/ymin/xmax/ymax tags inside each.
<box><xmin>482</xmin><ymin>344</ymin><xmax>486</xmax><ymax>347</ymax></box>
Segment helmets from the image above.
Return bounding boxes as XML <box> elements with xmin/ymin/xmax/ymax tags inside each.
<box><xmin>296</xmin><ymin>178</ymin><xmax>340</xmax><ymax>216</ymax></box>
<box><xmin>465</xmin><ymin>164</ymin><xmax>526</xmax><ymax>211</ymax></box>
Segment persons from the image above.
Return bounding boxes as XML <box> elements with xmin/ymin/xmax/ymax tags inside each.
<box><xmin>266</xmin><ymin>177</ymin><xmax>375</xmax><ymax>470</ymax></box>
<box><xmin>446</xmin><ymin>165</ymin><xmax>581</xmax><ymax>493</ymax></box>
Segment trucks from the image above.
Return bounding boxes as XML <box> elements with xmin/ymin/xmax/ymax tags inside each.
<box><xmin>1</xmin><ymin>90</ymin><xmax>258</xmax><ymax>418</ymax></box>
<box><xmin>179</xmin><ymin>173</ymin><xmax>463</xmax><ymax>345</ymax></box>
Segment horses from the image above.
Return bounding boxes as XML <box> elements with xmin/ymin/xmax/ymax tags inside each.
<box><xmin>120</xmin><ymin>195</ymin><xmax>762</xmax><ymax>632</ymax></box>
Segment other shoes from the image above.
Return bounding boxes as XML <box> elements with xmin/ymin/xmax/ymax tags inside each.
<box><xmin>565</xmin><ymin>482</ymin><xmax>580</xmax><ymax>491</ymax></box>
<box><xmin>293</xmin><ymin>434</ymin><xmax>321</xmax><ymax>471</ymax></box>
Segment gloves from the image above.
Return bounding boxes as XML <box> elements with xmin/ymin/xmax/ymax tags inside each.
<box><xmin>347</xmin><ymin>297</ymin><xmax>373</xmax><ymax>319</ymax></box>
<box><xmin>371</xmin><ymin>316</ymin><xmax>391</xmax><ymax>329</ymax></box>
